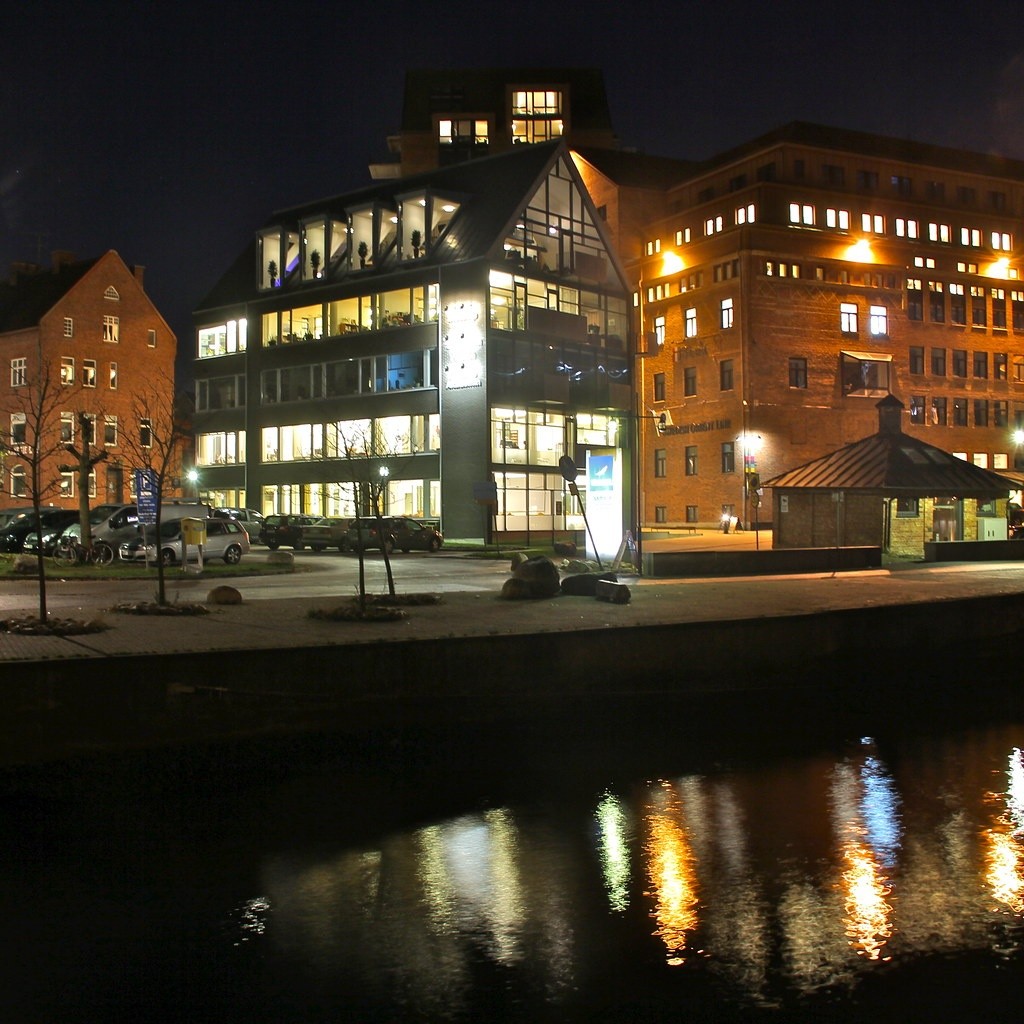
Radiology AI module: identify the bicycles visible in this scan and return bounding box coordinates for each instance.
[52,530,114,567]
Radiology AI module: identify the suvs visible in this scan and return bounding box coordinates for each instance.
[347,516,444,554]
[259,513,327,550]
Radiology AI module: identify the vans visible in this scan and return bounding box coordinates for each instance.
[211,507,268,545]
[118,516,251,566]
[1,506,59,533]
[60,502,210,562]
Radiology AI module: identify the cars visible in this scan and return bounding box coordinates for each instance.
[299,518,358,554]
[21,515,86,556]
[0,509,78,554]
